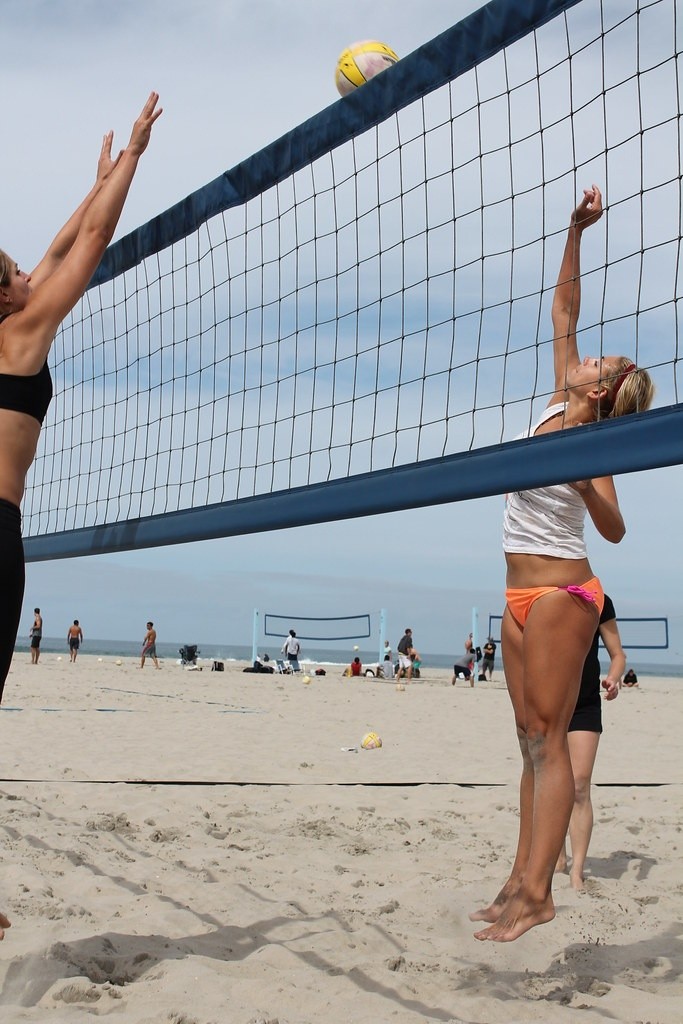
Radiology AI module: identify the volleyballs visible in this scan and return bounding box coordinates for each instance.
[333,38,401,98]
[56,656,63,662]
[360,731,383,751]
[115,659,122,666]
[301,675,311,685]
[353,645,360,651]
[97,657,103,662]
[395,684,405,692]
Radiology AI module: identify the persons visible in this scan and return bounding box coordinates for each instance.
[0,92,163,939]
[281,630,300,660]
[351,657,362,677]
[377,641,394,678]
[256,654,269,664]
[67,620,83,662]
[622,669,638,687]
[395,628,421,682]
[136,622,161,669]
[28,608,43,664]
[483,637,497,680]
[315,667,326,675]
[452,633,477,687]
[467,183,654,943]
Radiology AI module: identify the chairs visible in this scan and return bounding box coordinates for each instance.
[179,643,201,670]
[287,659,305,675]
[273,658,294,675]
